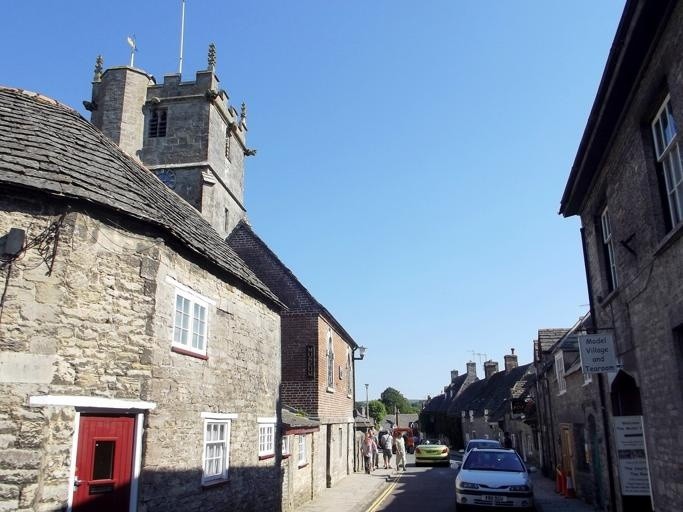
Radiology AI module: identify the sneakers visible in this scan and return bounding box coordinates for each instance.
[403,467,407,471]
[365,464,379,474]
[383,464,393,469]
[397,468,400,471]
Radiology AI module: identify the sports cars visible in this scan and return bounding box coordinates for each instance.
[414,439,450,467]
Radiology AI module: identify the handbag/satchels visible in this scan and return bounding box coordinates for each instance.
[381,444,384,449]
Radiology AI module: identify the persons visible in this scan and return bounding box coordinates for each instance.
[381,431,394,469]
[360,426,379,474]
[395,432,407,471]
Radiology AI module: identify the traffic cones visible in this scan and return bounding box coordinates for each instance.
[566,472,577,499]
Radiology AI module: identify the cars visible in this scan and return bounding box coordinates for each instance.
[449,446,537,512]
[459,439,502,463]
